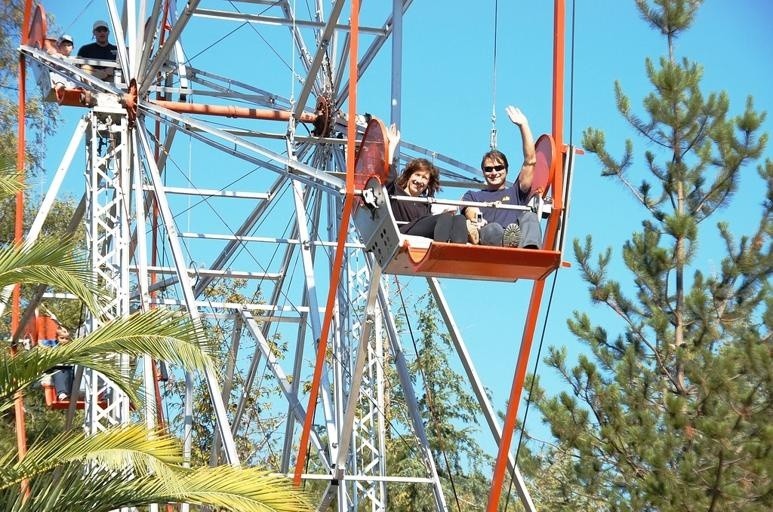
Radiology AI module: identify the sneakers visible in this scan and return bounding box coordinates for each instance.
[523,243,539,249]
[503,223,521,249]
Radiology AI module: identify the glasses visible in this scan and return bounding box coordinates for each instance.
[482,165,505,172]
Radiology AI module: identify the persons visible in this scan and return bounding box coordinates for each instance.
[77,19,129,90]
[44,34,92,105]
[460,105,541,250]
[383,123,467,243]
[41,324,74,401]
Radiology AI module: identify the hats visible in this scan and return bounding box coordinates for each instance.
[93,21,109,32]
[57,34,74,46]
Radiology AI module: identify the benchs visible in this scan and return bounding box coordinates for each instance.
[32,339,108,409]
[352,175,562,283]
[34,42,142,109]
